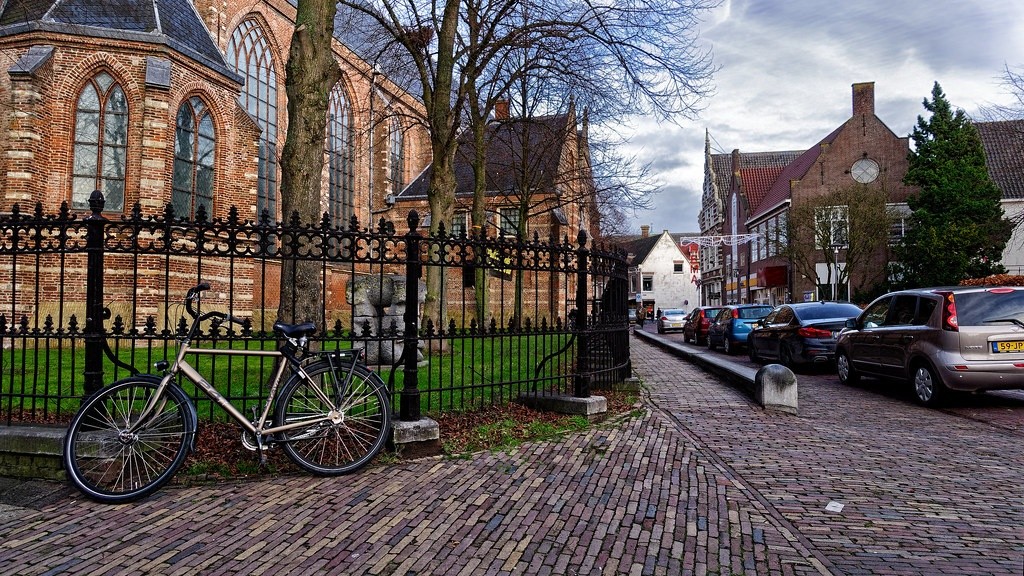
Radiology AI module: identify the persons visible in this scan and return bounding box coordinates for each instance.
[636,303,646,330]
[655,307,661,321]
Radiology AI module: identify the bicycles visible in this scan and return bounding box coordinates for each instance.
[63,282,392,505]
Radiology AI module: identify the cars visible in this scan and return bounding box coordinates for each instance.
[656,309,688,334]
[746,299,869,373]
[628,308,638,322]
[835,286,1024,410]
[683,306,729,347]
[707,303,777,357]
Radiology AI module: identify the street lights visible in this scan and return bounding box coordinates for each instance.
[830,241,845,302]
